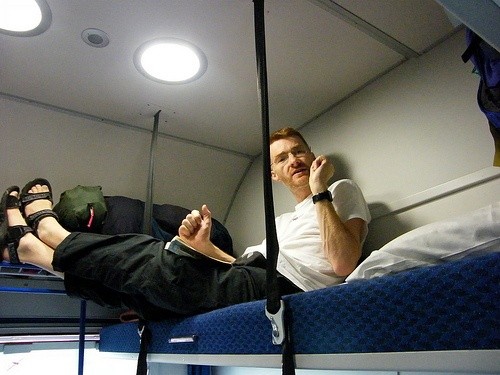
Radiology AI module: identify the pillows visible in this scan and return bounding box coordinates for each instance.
[345,201,500,282]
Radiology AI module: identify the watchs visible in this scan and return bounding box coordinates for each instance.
[312,190,333,204]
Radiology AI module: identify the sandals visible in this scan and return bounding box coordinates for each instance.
[0,185,34,266]
[19,178,60,231]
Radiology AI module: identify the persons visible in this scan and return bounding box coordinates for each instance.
[0,127,371,321]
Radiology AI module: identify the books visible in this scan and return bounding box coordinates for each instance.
[164,236,232,265]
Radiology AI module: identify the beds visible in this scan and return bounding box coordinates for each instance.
[99,251,500,371]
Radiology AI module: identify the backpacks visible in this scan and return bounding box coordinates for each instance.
[53,185,234,256]
